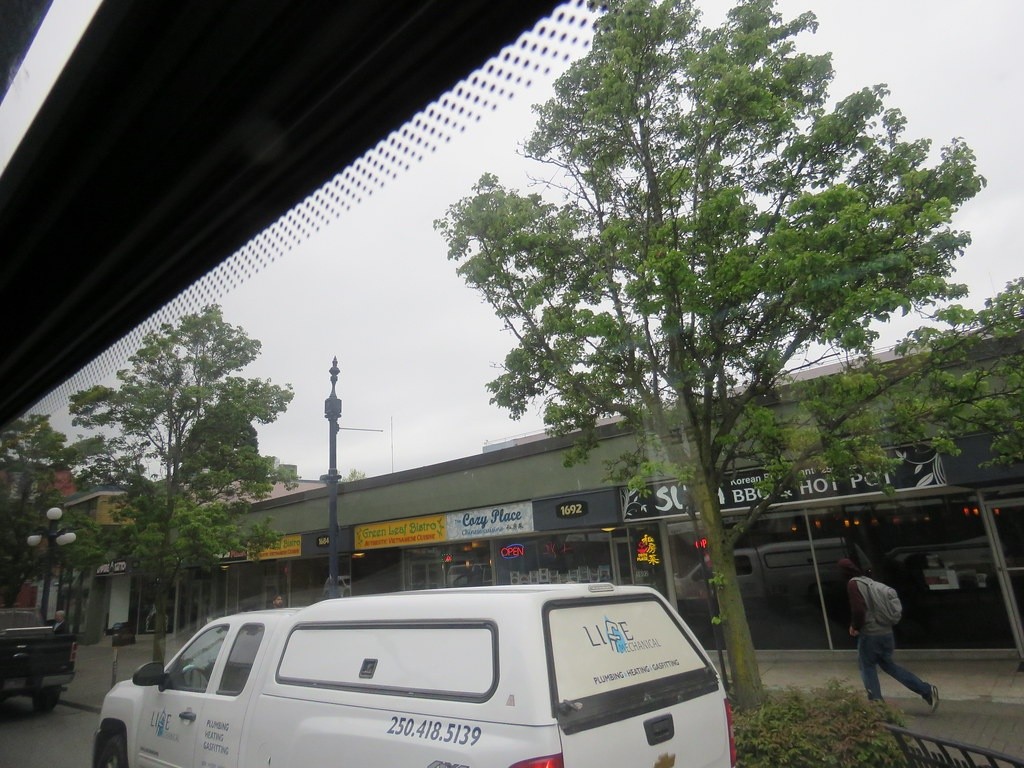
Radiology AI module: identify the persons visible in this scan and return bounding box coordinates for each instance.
[840,559,941,713]
[51,611,66,636]
[269,595,286,609]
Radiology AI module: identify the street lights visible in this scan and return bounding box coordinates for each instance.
[27,507,76,625]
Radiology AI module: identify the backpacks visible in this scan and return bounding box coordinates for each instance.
[849,576,902,626]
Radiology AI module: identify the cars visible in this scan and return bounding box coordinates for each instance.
[447,563,491,587]
[323,576,350,599]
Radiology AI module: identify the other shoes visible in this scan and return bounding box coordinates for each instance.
[62,687,67,691]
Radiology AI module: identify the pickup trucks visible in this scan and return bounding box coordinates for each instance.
[674,538,872,625]
[93,583,737,768]
[0,607,79,715]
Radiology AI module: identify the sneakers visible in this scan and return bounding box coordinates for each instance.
[929,685,940,713]
